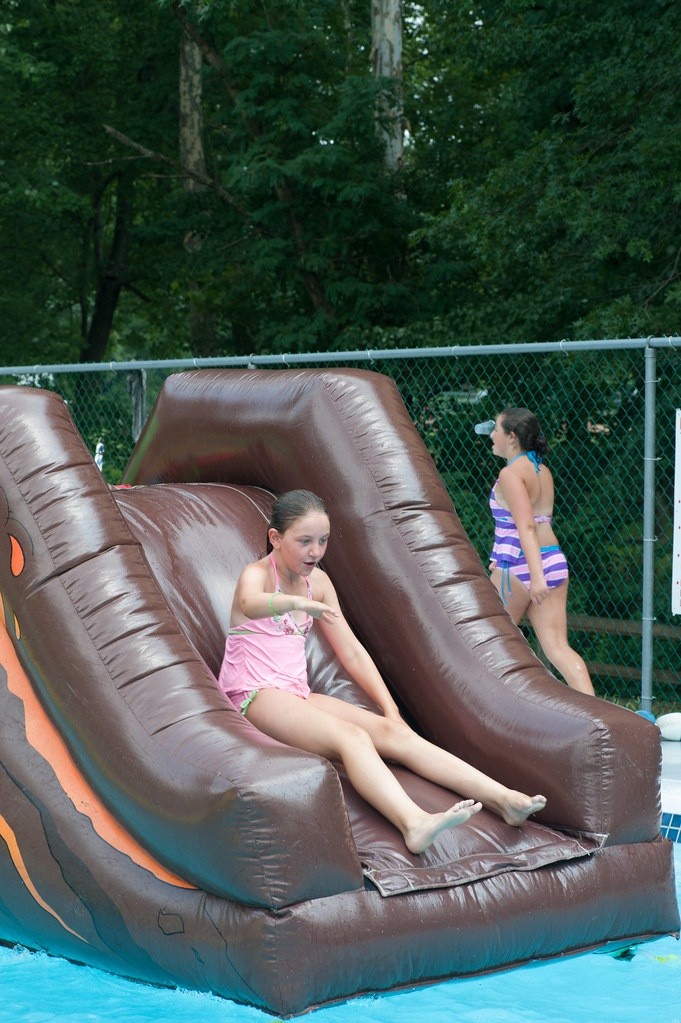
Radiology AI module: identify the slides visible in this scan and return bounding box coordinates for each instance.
[2,370,678,1014]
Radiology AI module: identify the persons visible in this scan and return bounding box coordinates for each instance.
[485,405,596,699]
[212,488,547,857]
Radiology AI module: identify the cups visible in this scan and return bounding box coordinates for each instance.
[474,419,494,435]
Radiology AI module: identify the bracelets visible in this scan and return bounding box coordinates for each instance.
[267,591,286,622]
